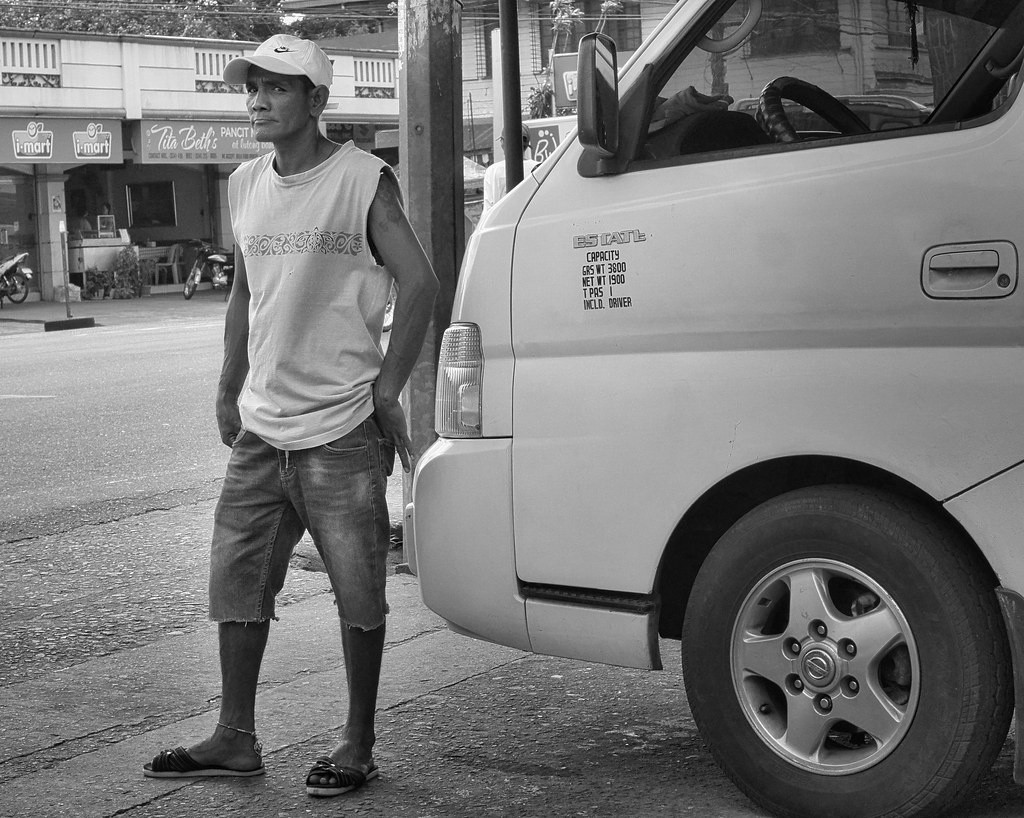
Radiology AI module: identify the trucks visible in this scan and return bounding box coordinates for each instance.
[404,0,1024,818]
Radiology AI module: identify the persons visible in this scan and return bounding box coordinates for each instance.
[484,123,541,211]
[142,33,439,795]
[71,202,111,231]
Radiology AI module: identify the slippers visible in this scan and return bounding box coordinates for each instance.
[142,746,265,777]
[306,755,379,795]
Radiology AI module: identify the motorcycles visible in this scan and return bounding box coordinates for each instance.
[183,236,232,300]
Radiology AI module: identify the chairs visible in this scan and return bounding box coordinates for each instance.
[156,243,183,284]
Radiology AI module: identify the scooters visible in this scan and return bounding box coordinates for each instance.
[0,250,34,305]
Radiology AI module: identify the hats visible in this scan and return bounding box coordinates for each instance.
[223,35,333,90]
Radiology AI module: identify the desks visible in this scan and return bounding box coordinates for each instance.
[139,246,184,284]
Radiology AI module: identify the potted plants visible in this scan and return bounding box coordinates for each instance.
[85,245,168,300]
